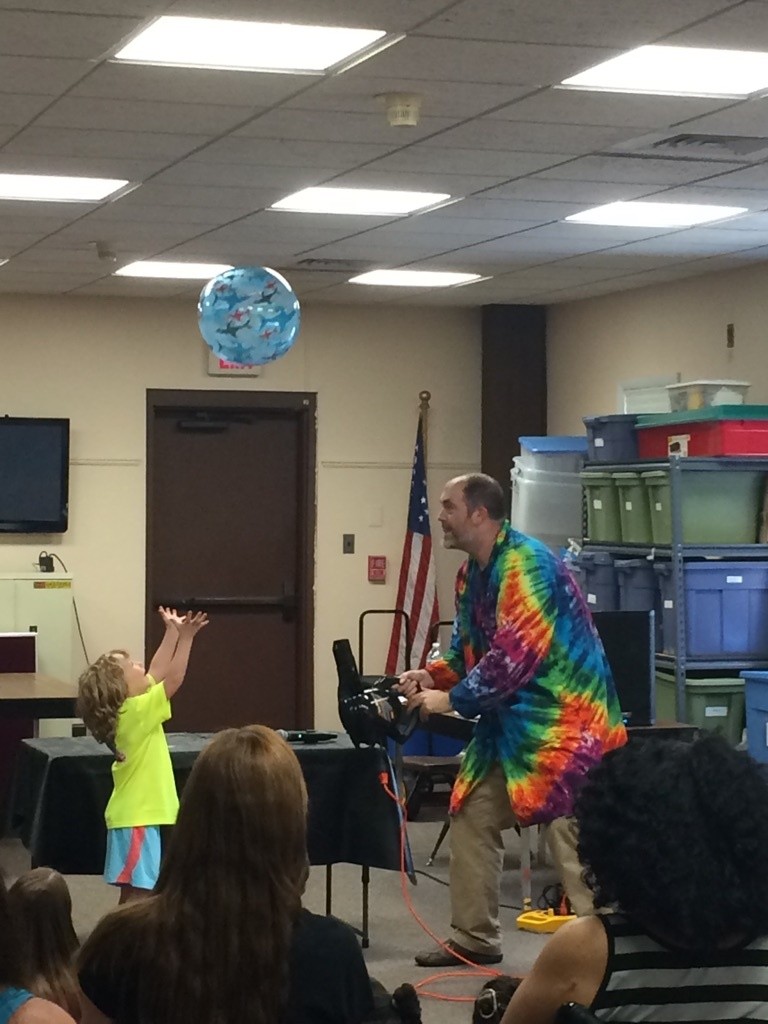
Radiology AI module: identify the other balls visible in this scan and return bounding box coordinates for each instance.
[195,266,307,367]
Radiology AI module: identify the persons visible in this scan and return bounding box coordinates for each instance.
[76,605,210,906]
[0,709,768,1024]
[391,474,627,969]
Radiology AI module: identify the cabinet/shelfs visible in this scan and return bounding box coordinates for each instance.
[579,450,768,723]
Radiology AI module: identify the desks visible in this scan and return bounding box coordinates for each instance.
[0,673,78,837]
[13,731,417,949]
[363,675,474,793]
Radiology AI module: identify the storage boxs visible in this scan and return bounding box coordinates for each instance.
[511,378,768,762]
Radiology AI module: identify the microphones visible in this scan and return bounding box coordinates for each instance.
[273,729,338,744]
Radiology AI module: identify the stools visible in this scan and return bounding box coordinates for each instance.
[400,754,463,865]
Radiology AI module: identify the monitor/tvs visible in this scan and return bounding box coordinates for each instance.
[0,417,69,531]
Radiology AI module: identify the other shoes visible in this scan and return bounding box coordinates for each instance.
[415,938,502,967]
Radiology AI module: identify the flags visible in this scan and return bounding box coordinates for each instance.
[382,413,443,680]
